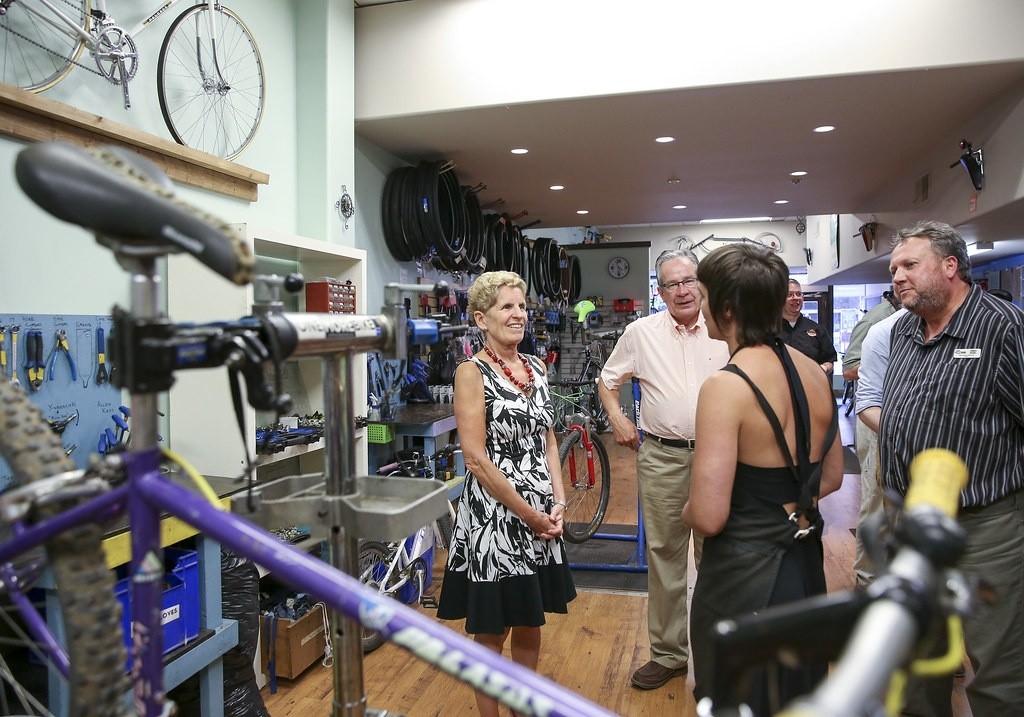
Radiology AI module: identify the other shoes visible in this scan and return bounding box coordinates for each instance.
[630,660,688,689]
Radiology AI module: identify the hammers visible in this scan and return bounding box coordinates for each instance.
[367,356,374,392]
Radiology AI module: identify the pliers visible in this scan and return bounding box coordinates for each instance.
[49,329,77,381]
[24,331,46,392]
[375,370,385,397]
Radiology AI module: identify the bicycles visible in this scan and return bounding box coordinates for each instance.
[838,351,859,418]
[326,443,462,653]
[0,0,267,166]
[0,135,975,716]
[547,378,611,544]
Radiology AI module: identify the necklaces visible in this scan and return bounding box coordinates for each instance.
[482,344,536,392]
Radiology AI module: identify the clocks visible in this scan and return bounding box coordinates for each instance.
[608,257,630,279]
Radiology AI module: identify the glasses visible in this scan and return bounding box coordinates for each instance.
[662,278,698,291]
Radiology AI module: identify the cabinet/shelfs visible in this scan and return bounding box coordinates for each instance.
[166,225,369,690]
[8,470,265,717]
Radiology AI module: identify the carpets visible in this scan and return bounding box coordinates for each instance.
[563,522,647,592]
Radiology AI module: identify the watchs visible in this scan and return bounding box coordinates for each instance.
[554,500,569,513]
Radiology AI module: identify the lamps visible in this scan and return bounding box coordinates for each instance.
[967,243,994,256]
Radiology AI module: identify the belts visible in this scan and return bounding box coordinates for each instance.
[648,434,694,450]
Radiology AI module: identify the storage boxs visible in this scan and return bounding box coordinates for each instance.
[114,545,198,676]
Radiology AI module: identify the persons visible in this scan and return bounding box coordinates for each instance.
[678,241,847,717]
[842,283,914,588]
[781,276,838,375]
[597,247,732,692]
[449,268,569,717]
[875,218,1024,717]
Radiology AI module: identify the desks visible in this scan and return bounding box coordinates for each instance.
[391,401,466,501]
